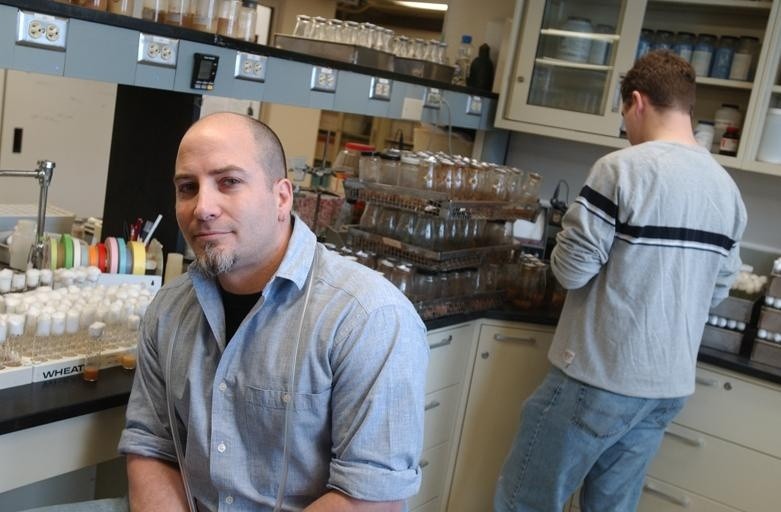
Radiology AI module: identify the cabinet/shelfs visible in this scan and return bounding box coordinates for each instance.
[569,363,781,512]
[446,323,558,511]
[326,178,542,324]
[412,321,478,511]
[493,0,781,177]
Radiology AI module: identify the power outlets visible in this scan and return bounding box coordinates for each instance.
[233,50,268,82]
[424,86,447,109]
[368,76,394,101]
[311,66,339,92]
[14,9,70,51]
[137,34,181,67]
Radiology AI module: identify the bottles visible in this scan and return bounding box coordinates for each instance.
[292,14,447,66]
[451,35,493,95]
[323,241,547,302]
[694,103,744,157]
[359,204,517,252]
[555,15,761,82]
[52,0,257,45]
[0,265,157,382]
[358,148,543,204]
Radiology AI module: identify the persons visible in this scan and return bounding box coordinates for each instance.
[117,112,430,512]
[492,49,748,512]
